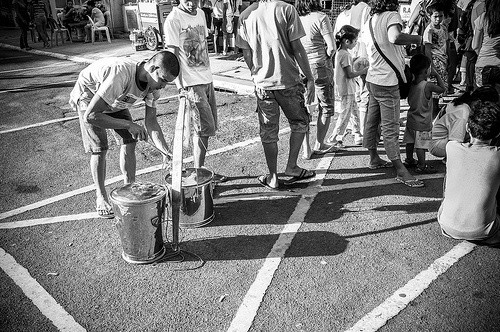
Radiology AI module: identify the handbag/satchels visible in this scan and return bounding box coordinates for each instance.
[396,64,413,99]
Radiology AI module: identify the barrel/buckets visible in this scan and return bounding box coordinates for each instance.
[165,168,215,229]
[110,183,166,263]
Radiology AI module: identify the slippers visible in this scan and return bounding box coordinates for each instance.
[283,168,316,185]
[395,173,425,188]
[95,203,115,219]
[314,144,340,154]
[367,160,394,170]
[257,175,279,190]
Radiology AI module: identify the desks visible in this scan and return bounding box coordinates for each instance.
[68,22,88,42]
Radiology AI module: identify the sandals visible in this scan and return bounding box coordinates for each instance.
[403,158,418,168]
[413,164,439,175]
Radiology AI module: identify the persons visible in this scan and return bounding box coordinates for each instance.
[164,1,229,184]
[437,100,500,240]
[68,52,180,220]
[236,0,316,189]
[13,0,500,188]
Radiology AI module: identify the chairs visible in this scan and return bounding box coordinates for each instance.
[47,17,73,47]
[87,15,111,46]
